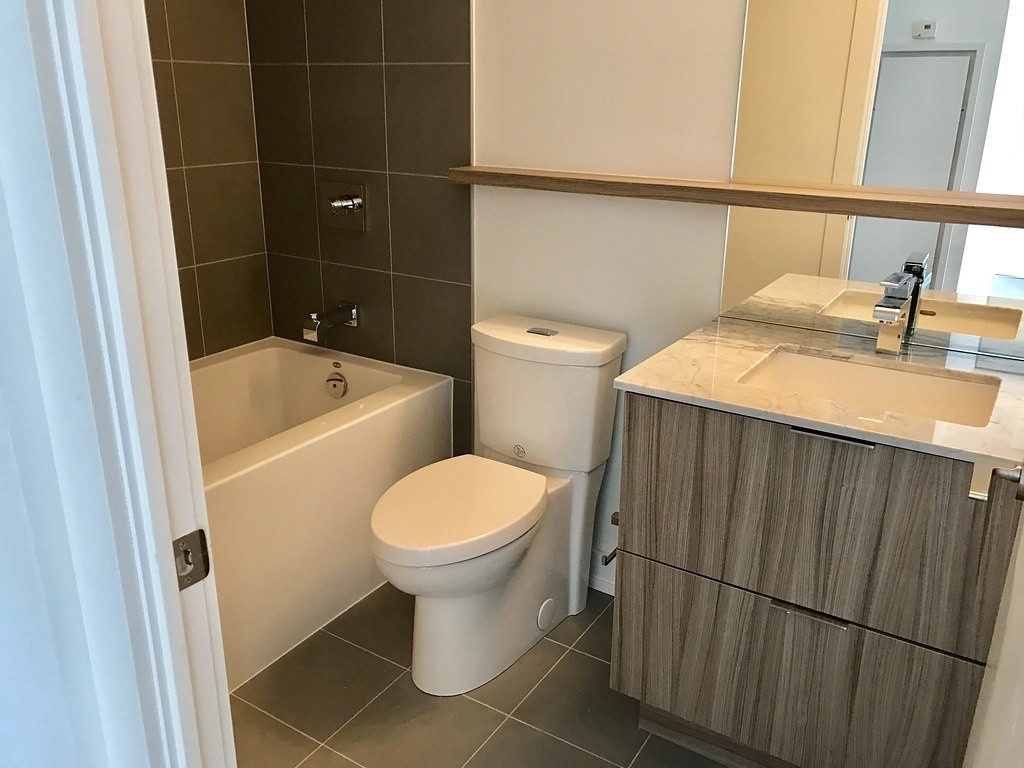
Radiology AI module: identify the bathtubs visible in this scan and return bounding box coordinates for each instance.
[188,335,453,696]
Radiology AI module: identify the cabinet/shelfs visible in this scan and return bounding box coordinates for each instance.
[610,389,1024,768]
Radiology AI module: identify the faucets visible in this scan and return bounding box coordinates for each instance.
[900,252,933,336]
[302,300,359,343]
[872,271,914,356]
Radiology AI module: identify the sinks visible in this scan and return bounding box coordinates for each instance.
[734,343,1002,428]
[816,288,1022,341]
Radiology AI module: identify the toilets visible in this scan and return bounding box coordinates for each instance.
[369,312,627,697]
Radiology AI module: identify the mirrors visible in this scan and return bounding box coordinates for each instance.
[718,1,1023,362]
